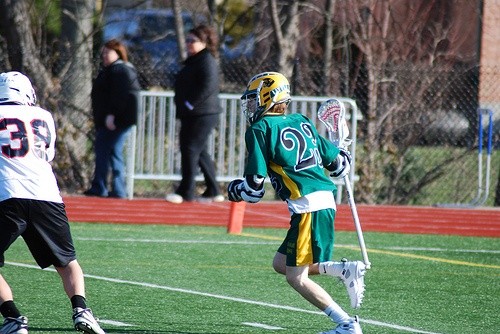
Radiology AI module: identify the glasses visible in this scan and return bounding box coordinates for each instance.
[186,39,202,43]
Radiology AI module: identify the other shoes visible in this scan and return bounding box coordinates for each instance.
[166,193,184,204]
[108,191,118,197]
[83,188,98,196]
[196,194,224,203]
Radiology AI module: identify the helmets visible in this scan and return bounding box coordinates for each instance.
[241,72,291,125]
[0,72,37,106]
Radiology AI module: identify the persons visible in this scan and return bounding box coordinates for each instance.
[84,38,139,201]
[0,72,105,334]
[165,24,225,204]
[228,71,366,334]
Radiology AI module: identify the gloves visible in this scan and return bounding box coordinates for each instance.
[227,180,243,202]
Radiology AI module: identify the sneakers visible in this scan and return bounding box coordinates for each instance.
[318,315,362,334]
[72,307,105,334]
[341,258,365,310]
[0,316,29,334]
